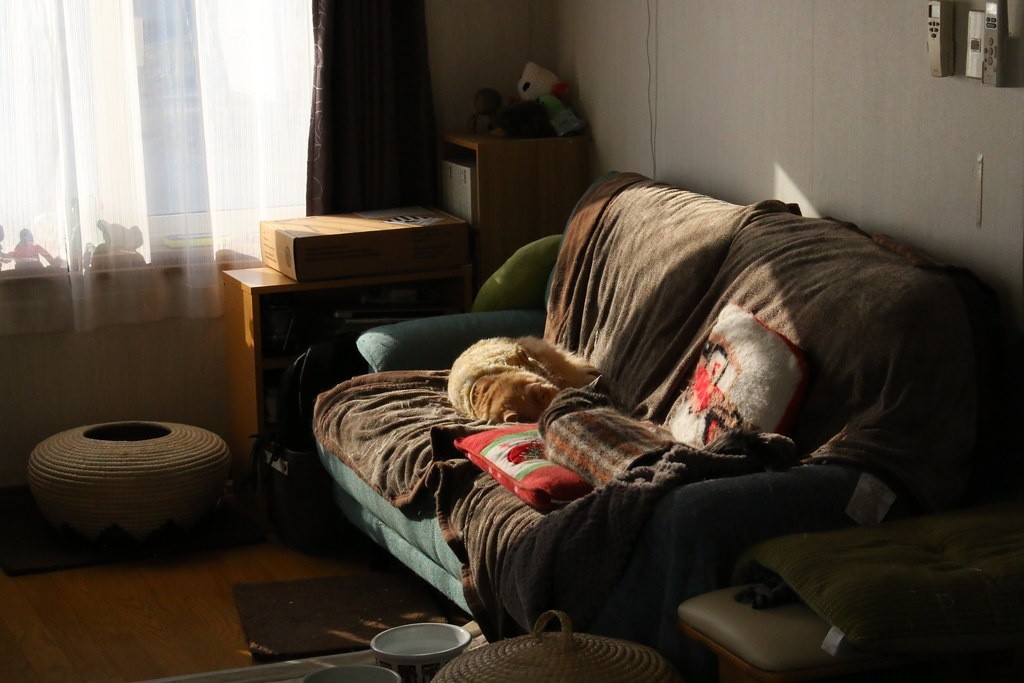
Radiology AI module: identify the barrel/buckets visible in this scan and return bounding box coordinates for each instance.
[264,433,340,556]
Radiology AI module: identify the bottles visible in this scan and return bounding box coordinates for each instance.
[261,302,300,357]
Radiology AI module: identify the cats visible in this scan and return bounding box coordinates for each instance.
[538,374,768,489]
[447,334,605,426]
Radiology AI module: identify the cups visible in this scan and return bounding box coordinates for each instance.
[370,622,472,683]
[302,664,402,683]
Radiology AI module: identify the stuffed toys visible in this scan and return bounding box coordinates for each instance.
[516,58,570,103]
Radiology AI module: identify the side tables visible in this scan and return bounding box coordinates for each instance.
[221,127,587,487]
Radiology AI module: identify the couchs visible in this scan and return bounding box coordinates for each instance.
[297,171,1011,629]
